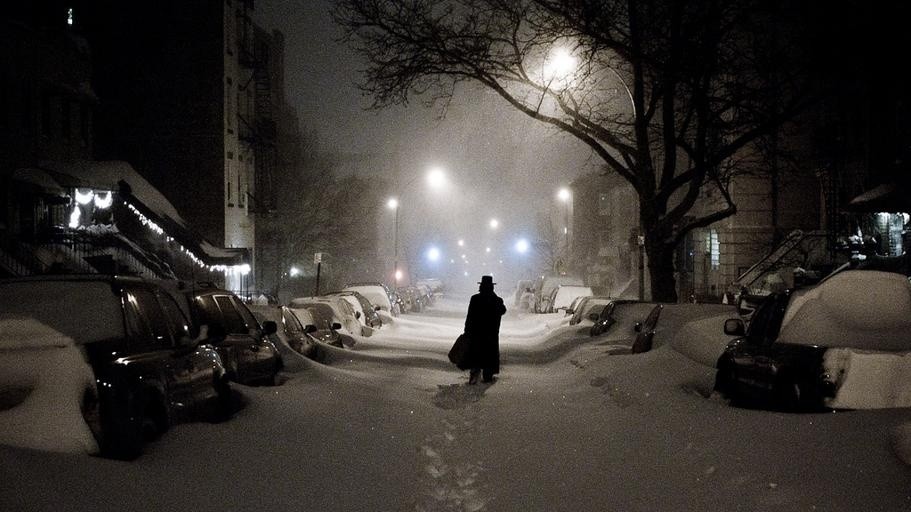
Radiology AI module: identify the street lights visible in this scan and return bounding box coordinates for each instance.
[385,166,446,292]
[557,188,573,267]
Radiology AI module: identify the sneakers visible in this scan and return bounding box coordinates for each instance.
[468,369,498,383]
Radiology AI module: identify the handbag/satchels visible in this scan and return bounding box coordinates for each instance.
[449,332,473,371]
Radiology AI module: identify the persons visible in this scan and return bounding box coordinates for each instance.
[462,275,508,385]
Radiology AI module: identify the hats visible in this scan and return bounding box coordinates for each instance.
[477,275,498,286]
